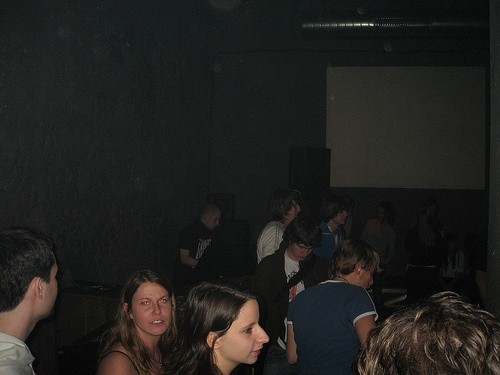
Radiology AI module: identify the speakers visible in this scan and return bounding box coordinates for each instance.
[288,146,331,193]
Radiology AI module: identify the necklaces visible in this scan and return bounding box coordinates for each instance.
[151,356,164,366]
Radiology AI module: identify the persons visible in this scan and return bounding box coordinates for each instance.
[164,282,269,375]
[96,265,177,375]
[250,216,333,375]
[0,226,59,375]
[175,186,500,311]
[286,237,380,375]
